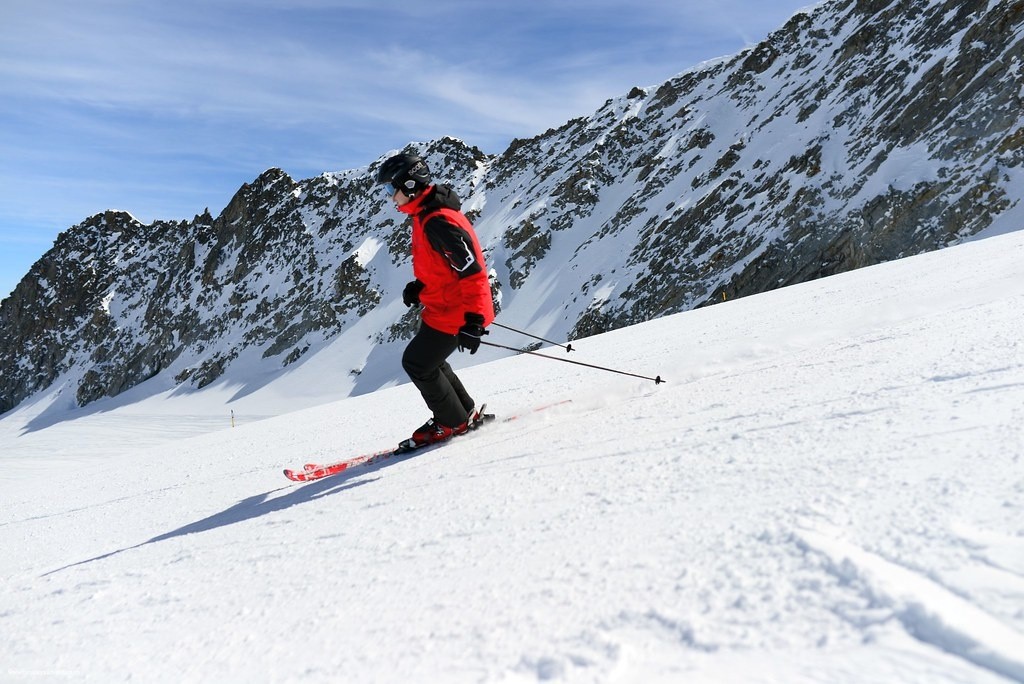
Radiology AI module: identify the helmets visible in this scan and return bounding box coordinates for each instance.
[376,152,432,185]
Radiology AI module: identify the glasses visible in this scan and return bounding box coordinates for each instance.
[385,179,397,197]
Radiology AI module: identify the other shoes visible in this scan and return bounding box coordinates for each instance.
[411,410,479,443]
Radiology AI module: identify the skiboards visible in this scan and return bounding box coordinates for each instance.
[281,398,572,480]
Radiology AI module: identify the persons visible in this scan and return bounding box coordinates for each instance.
[373,153,499,451]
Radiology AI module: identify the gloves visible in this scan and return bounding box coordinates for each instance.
[457,313,487,353]
[402,280,424,306]
